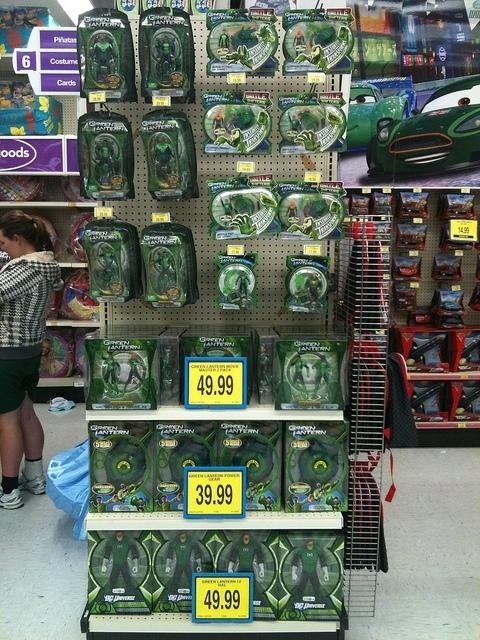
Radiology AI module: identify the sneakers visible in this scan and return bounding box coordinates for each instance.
[18,468,48,495]
[0,481,26,510]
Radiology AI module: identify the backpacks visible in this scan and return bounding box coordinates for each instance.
[352,345,420,453]
[347,217,393,332]
[342,458,391,575]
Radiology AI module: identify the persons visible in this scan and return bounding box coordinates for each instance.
[90,31,177,85]
[101,532,329,606]
[92,242,176,296]
[92,134,176,188]
[74,4,353,622]
[101,344,331,396]
[0,209,66,511]
[212,26,321,303]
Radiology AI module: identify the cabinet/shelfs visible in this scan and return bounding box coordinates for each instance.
[339,190,479,452]
[0,0,93,396]
[77,4,350,640]
